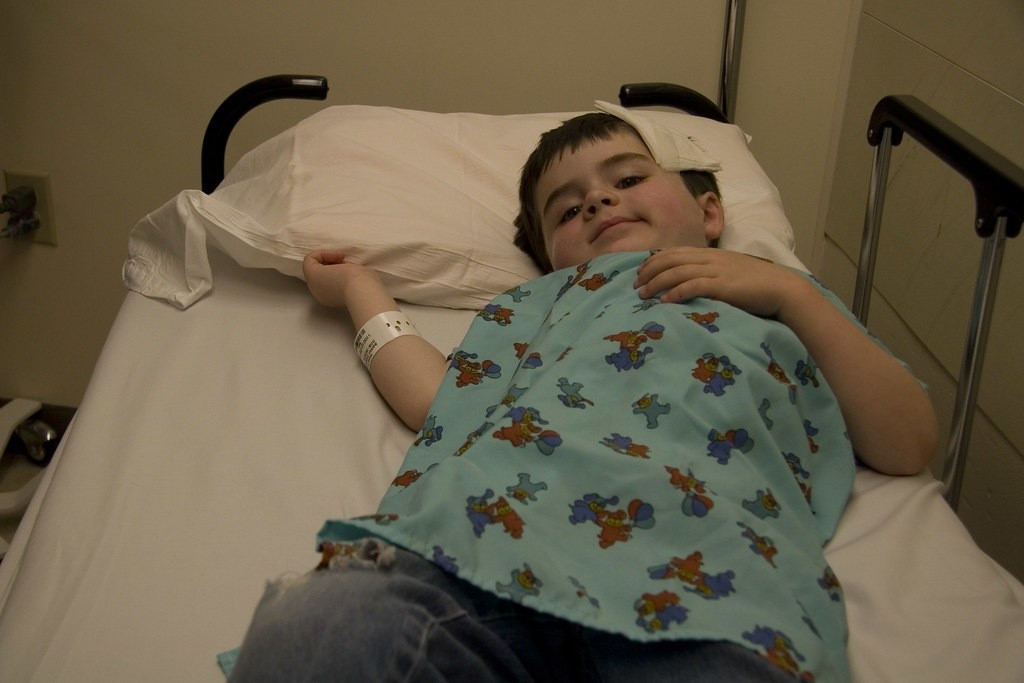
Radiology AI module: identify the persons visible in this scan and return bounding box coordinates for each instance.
[228,113,937,683]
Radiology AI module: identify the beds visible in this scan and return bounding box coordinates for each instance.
[0,77,1024,683]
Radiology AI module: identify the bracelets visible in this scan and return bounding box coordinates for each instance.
[354,311,421,372]
